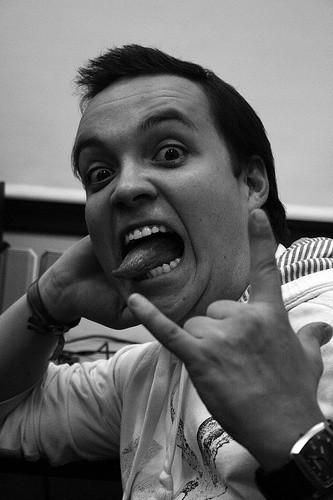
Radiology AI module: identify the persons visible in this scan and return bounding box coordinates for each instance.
[0,41,333,500]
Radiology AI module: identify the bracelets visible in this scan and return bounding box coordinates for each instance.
[22,280,81,339]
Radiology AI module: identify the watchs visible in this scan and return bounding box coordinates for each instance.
[252,415,333,500]
